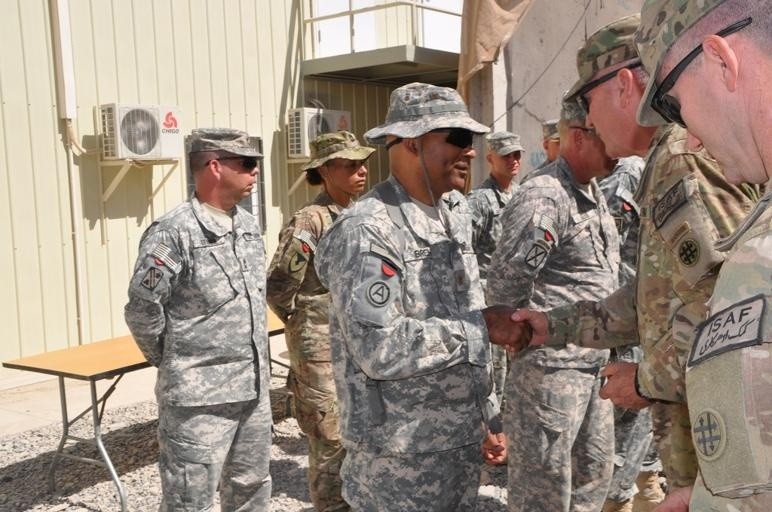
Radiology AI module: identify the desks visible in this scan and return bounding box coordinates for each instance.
[1,304,293,512]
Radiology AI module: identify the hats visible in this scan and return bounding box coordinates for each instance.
[634,0,729,128]
[301,131,376,170]
[561,14,643,103]
[486,132,526,156]
[364,83,491,144]
[542,121,559,139]
[190,128,264,158]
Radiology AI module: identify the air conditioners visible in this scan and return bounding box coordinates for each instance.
[100,100,186,161]
[287,106,352,159]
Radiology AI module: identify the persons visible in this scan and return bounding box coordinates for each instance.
[520,120,561,184]
[597,154,664,512]
[633,0,772,512]
[504,12,762,494]
[313,81,534,512]
[124,128,272,512]
[480,90,622,511]
[466,129,527,409]
[266,130,376,512]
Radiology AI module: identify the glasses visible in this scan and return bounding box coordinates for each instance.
[205,156,257,168]
[649,17,755,128]
[576,62,642,116]
[445,129,473,148]
